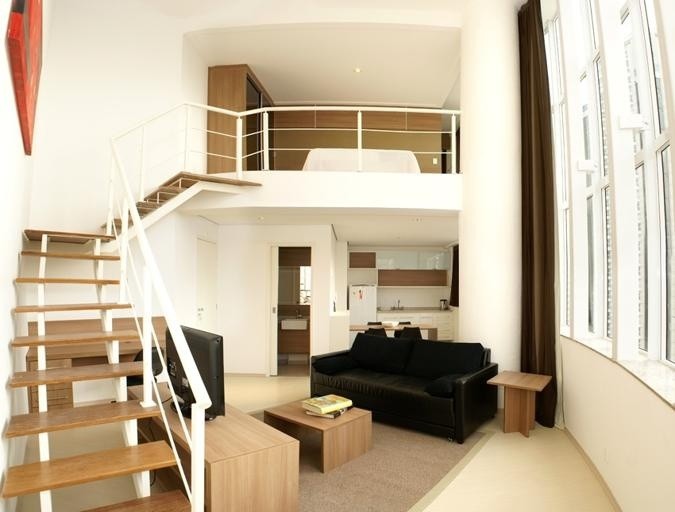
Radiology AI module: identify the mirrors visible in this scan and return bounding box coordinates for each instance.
[279,265,312,306]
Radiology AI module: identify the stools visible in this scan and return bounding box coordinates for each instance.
[487,370,553,440]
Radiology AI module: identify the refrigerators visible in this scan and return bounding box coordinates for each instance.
[349,283,377,348]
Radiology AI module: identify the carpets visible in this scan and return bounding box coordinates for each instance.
[245,396,496,511]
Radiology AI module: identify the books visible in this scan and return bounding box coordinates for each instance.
[301,393,354,414]
[305,405,353,420]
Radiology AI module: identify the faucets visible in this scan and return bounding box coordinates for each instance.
[398,299,400,308]
[296,309,302,319]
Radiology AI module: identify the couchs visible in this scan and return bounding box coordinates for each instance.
[310,332,498,444]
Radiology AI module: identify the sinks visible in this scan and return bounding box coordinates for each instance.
[281,319,308,331]
[377,307,403,311]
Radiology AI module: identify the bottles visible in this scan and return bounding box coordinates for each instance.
[439,298,448,310]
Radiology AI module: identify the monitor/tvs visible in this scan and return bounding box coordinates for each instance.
[165,325,225,422]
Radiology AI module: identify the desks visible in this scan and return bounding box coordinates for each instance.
[117,380,301,512]
